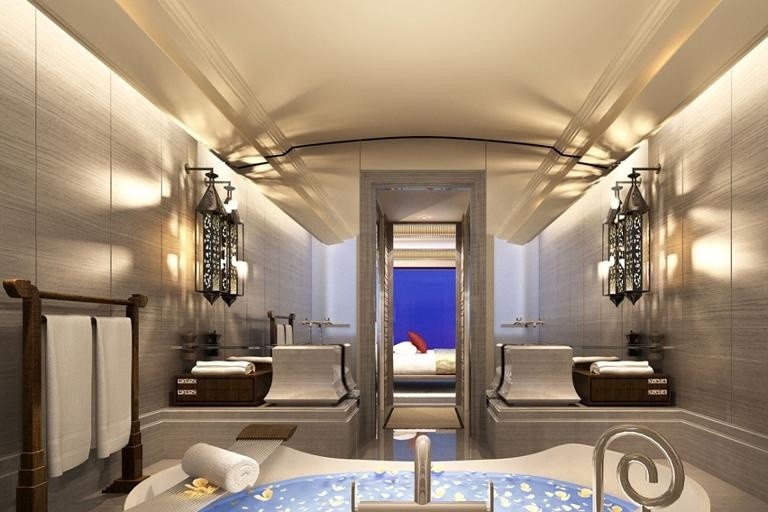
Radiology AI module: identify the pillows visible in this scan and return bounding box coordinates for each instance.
[407,331,428,353]
[392,341,417,353]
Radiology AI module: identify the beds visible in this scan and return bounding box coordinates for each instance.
[392,348,457,383]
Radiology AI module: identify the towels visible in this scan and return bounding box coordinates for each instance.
[276,324,285,347]
[181,441,260,494]
[44,315,92,478]
[572,356,622,368]
[226,356,273,363]
[284,324,294,346]
[589,360,654,374]
[93,316,133,459]
[190,361,255,375]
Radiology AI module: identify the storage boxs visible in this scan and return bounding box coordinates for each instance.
[173,369,273,406]
[572,369,672,407]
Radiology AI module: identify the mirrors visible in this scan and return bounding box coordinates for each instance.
[485,139,642,398]
[361,138,485,172]
[205,136,361,398]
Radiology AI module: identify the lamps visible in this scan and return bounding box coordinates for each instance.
[617,162,661,305]
[204,177,245,308]
[183,163,229,307]
[601,178,644,308]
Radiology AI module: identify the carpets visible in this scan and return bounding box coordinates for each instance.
[381,406,464,429]
